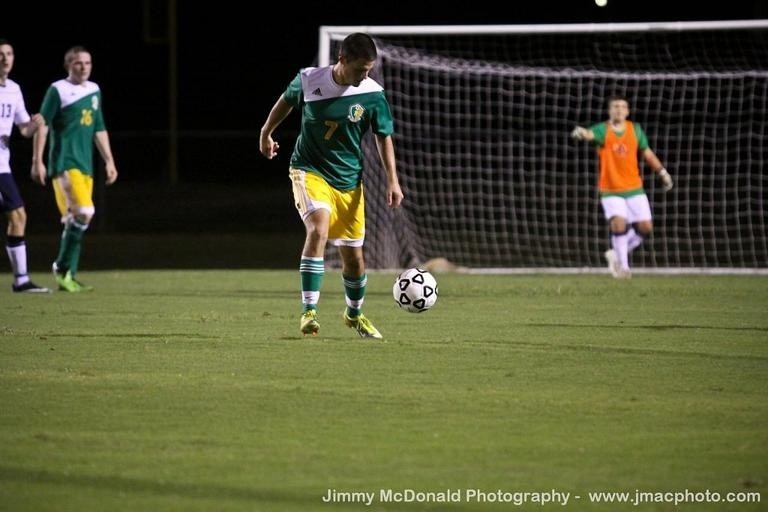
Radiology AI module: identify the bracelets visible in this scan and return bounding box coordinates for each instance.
[657,166,666,179]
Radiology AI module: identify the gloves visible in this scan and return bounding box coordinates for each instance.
[571,126,587,140]
[659,168,673,193]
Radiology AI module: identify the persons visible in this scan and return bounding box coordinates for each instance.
[259,32,404,342]
[28,43,121,296]
[0,40,58,295]
[568,94,674,280]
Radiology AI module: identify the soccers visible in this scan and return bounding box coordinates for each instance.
[392,266,438,312]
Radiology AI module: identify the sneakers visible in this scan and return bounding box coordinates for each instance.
[603,249,632,281]
[299,309,320,336]
[342,306,383,339]
[12,281,53,293]
[51,261,94,293]
